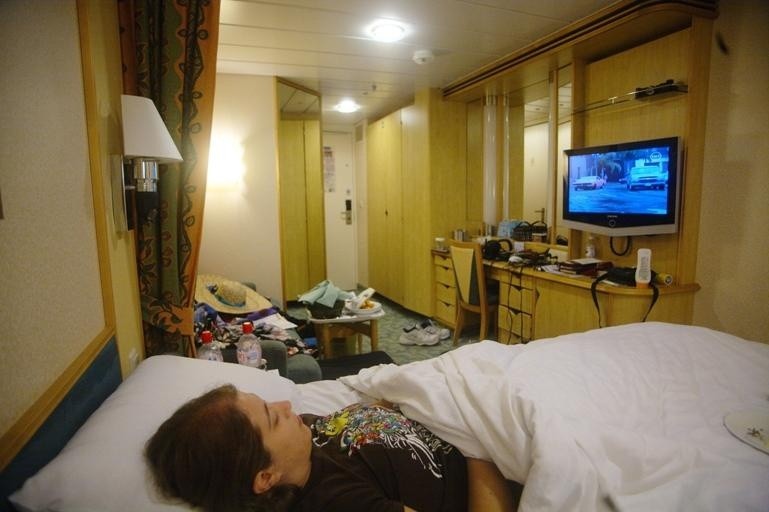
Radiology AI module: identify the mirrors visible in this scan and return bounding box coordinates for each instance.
[506,63,572,237]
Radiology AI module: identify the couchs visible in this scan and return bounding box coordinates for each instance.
[202,280,324,384]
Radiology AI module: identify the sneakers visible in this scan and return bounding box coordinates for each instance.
[398,326,440,346]
[416,318,451,340]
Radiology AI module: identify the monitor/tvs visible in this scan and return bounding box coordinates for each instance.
[557,136,680,237]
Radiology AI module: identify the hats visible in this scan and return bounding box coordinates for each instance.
[196,274,273,316]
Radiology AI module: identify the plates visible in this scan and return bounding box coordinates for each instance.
[346,303,382,314]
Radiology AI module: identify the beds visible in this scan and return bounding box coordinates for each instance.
[0,321,769,512]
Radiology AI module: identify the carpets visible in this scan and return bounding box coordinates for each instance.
[317,350,396,380]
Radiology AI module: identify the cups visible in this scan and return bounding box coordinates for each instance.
[434,237,445,251]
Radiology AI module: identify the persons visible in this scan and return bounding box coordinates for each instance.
[141,381,525,512]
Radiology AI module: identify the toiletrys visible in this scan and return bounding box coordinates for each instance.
[634,249,651,289]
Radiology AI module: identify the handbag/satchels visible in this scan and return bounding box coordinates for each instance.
[605,266,658,287]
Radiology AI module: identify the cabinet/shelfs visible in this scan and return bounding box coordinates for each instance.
[366,87,467,318]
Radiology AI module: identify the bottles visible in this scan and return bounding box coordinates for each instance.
[235,321,262,369]
[584,236,595,262]
[198,329,221,367]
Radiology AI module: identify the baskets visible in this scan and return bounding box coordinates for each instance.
[512,220,547,242]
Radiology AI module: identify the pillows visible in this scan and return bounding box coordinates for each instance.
[8,354,296,512]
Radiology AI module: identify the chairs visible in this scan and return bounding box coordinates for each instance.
[447,239,498,347]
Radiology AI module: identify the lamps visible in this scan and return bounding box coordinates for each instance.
[120,94,184,193]
[361,14,414,47]
[333,99,361,113]
[412,49,435,64]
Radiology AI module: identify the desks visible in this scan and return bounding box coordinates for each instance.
[431,248,701,346]
